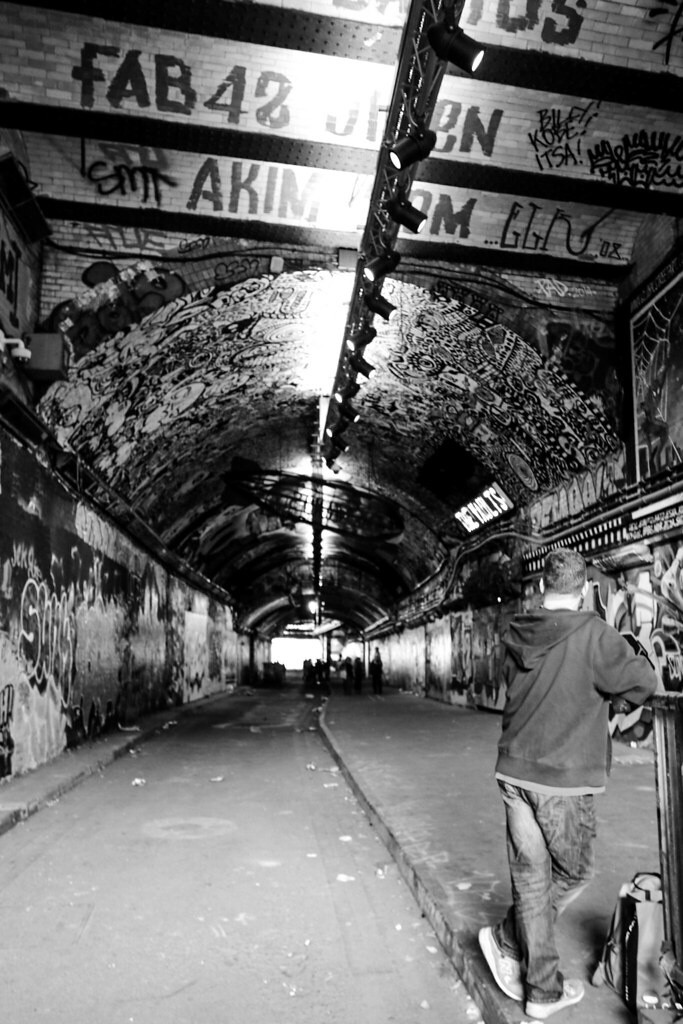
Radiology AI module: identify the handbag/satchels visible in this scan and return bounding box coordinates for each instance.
[593,873,669,1010]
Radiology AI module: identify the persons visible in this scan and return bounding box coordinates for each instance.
[478,549,656,1019]
[304,654,382,694]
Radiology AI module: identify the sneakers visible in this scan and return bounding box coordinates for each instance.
[479,927,525,1001]
[526,979,585,1020]
